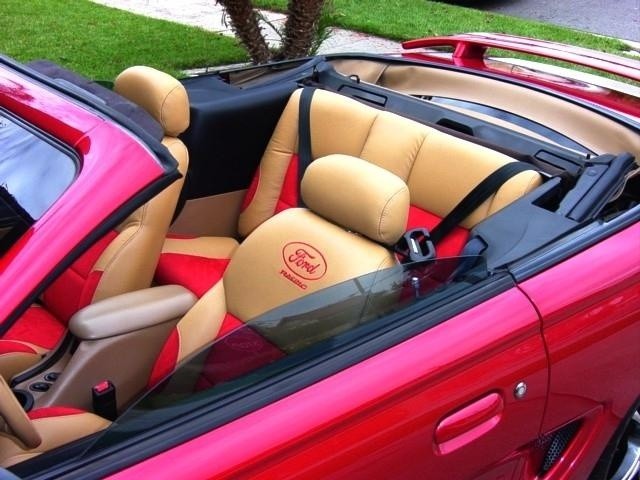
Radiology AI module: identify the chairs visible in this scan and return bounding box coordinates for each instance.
[0,155,411,467]
[0,64,191,373]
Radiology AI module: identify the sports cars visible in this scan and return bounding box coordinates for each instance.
[0,31,640,480]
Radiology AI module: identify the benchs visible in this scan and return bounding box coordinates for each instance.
[158,86,543,325]
